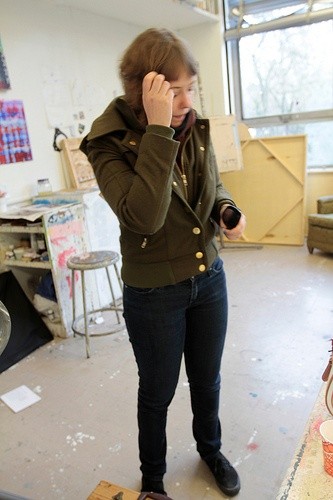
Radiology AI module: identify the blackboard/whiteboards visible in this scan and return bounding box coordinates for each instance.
[205,114,245,174]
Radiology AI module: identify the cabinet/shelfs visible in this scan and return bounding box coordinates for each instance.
[0,191,122,340]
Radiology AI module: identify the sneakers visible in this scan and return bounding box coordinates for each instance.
[198,451,240,496]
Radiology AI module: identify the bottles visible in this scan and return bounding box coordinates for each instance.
[36,179,53,196]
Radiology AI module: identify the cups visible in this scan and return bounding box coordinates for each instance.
[319,419,333,475]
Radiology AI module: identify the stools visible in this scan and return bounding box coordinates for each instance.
[66,251,127,359]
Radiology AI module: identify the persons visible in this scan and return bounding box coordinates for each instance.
[78,28,246,500]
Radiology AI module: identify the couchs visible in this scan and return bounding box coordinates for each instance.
[307,196,333,255]
